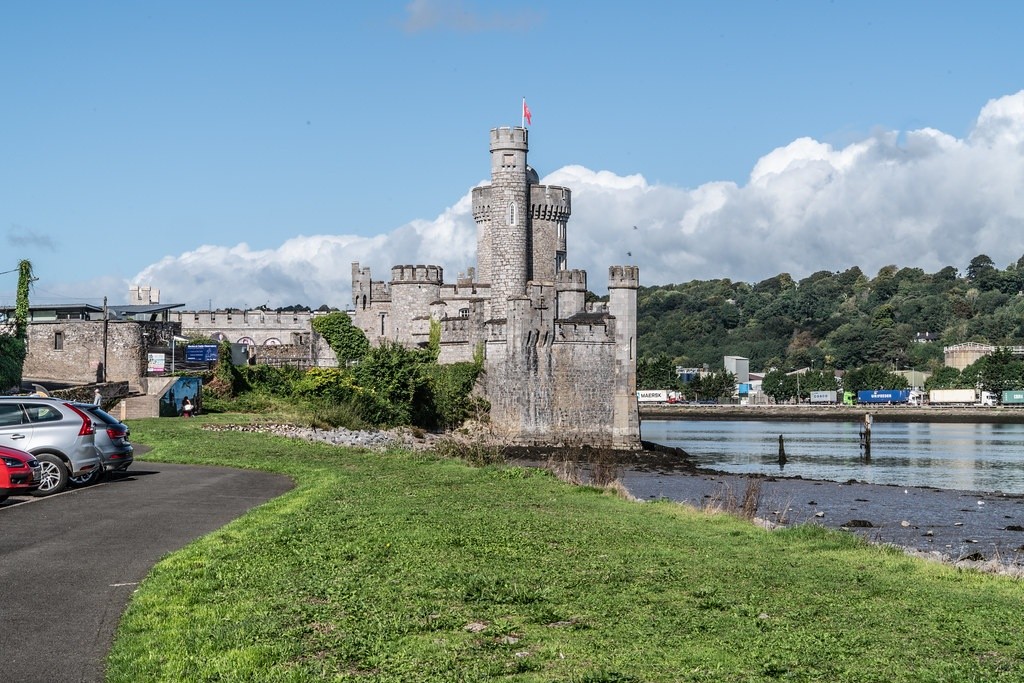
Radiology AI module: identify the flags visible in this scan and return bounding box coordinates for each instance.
[523,102,532,125]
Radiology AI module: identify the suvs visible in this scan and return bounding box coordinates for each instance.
[0,392,101,497]
[72,401,134,473]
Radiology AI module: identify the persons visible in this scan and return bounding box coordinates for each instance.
[169,389,200,418]
[93,389,102,409]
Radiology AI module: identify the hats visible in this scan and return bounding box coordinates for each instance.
[94,389,101,393]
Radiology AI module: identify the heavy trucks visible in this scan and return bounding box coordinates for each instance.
[636,389,682,404]
[1001,391,1024,409]
[857,390,919,408]
[928,388,996,407]
[808,389,857,405]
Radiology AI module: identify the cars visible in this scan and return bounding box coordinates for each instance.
[0,444,43,505]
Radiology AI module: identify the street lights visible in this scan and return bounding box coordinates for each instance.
[903,365,915,391]
[790,366,800,404]
